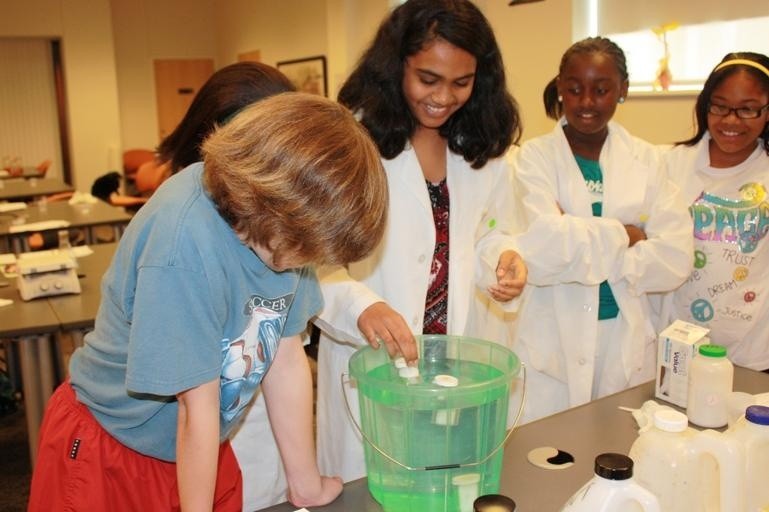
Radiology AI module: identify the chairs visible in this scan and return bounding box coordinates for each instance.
[122,150,174,195]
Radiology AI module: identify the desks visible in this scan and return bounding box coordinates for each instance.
[255,365,769,512]
[0,242,132,475]
[1,167,41,180]
[1,178,75,198]
[1,191,133,253]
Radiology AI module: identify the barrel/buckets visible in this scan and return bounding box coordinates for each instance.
[341,333,528,512]
[630,407,736,511]
[736,406,769,511]
[559,453,656,511]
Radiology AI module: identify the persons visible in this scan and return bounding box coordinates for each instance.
[505,37,699,427]
[646,49,769,375]
[302,1,528,482]
[160,59,312,511]
[25,89,390,512]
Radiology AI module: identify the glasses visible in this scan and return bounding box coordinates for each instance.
[708,100,769,119]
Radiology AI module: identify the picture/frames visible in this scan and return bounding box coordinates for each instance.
[278,55,328,96]
